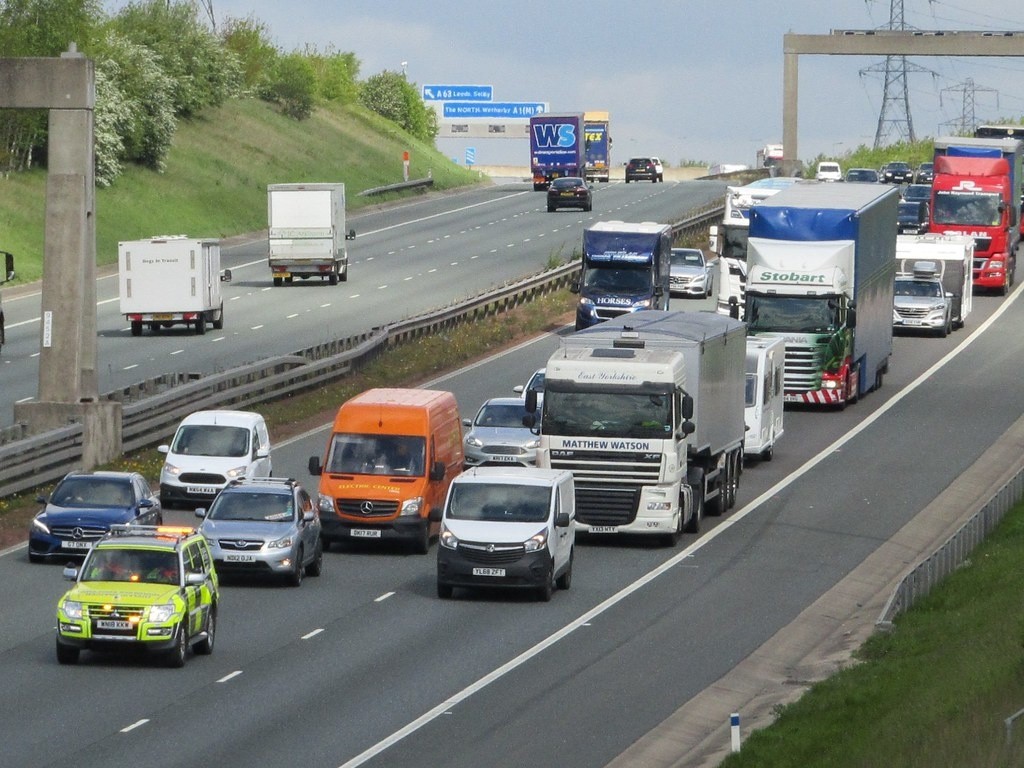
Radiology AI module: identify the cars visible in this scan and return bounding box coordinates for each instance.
[460,369,546,468]
[846,162,933,235]
[546,179,593,213]
[895,274,954,337]
[669,247,714,298]
[27,470,163,561]
[623,157,663,183]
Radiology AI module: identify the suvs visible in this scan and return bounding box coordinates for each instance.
[54,525,220,665]
[194,476,323,587]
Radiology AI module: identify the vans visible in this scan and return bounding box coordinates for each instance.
[308,386,462,555]
[157,410,271,507]
[815,162,842,181]
[430,468,576,600]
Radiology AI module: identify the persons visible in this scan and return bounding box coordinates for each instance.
[387,440,418,472]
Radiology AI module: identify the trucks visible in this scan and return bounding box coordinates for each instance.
[267,183,357,284]
[530,111,612,190]
[757,143,783,168]
[918,126,1024,295]
[118,234,232,333]
[572,219,671,332]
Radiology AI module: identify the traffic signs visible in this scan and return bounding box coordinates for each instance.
[422,85,546,118]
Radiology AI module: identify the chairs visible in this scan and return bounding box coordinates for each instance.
[102,553,140,581]
[492,406,509,424]
[645,160,650,164]
[142,552,180,586]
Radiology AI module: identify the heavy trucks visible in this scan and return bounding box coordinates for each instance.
[521,308,750,545]
[709,177,897,409]
